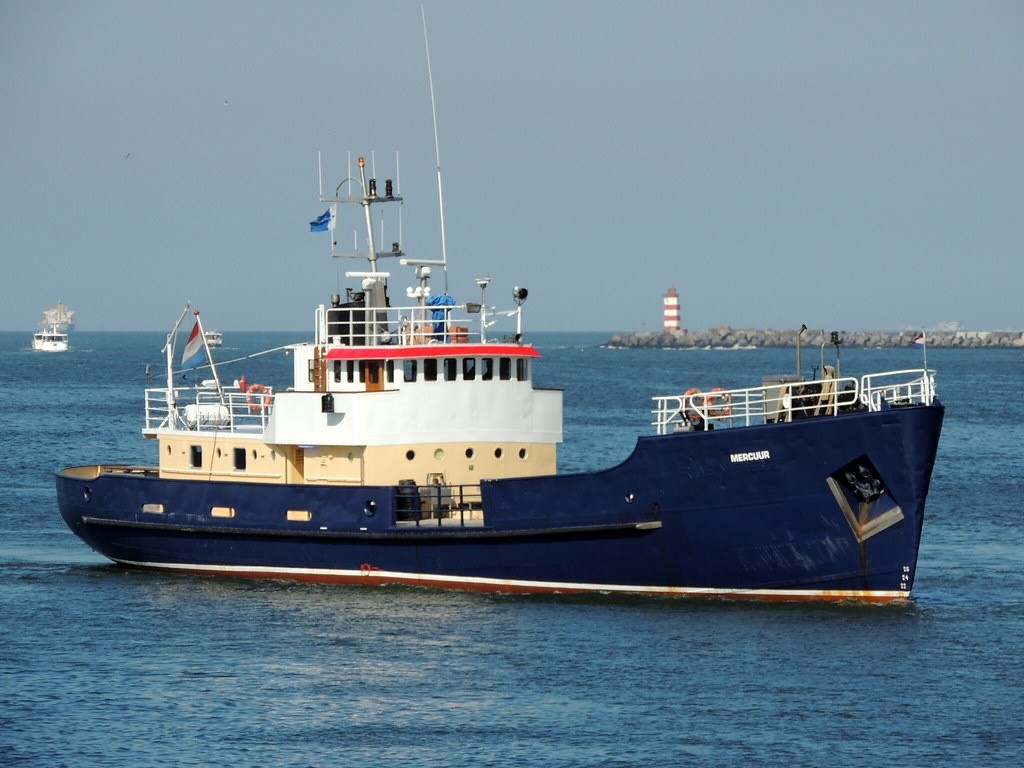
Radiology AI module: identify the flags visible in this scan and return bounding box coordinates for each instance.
[310,203,337,233]
[180,321,209,380]
[911,331,924,351]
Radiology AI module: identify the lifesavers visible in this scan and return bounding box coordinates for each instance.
[245,384,271,411]
[682,388,705,419]
[707,388,731,417]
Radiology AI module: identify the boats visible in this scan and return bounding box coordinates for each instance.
[44,304,75,335]
[34,327,67,353]
[204,330,223,347]
[55,145,944,609]
